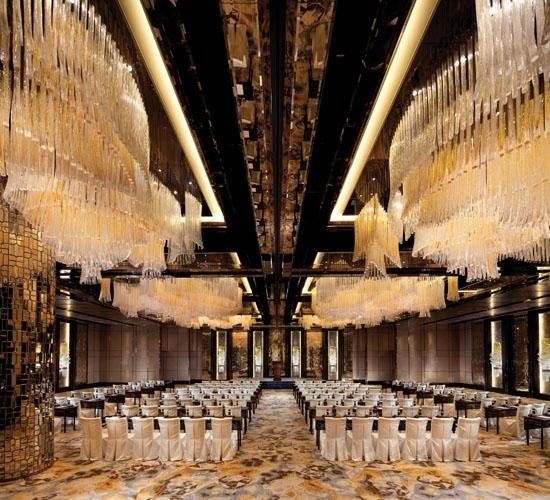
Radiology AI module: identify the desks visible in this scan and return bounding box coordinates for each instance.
[522,415,550,449]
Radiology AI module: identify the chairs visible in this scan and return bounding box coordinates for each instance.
[294,380,546,463]
[54,379,261,463]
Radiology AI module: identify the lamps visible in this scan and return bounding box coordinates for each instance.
[298,1,548,331]
[0,1,251,332]
